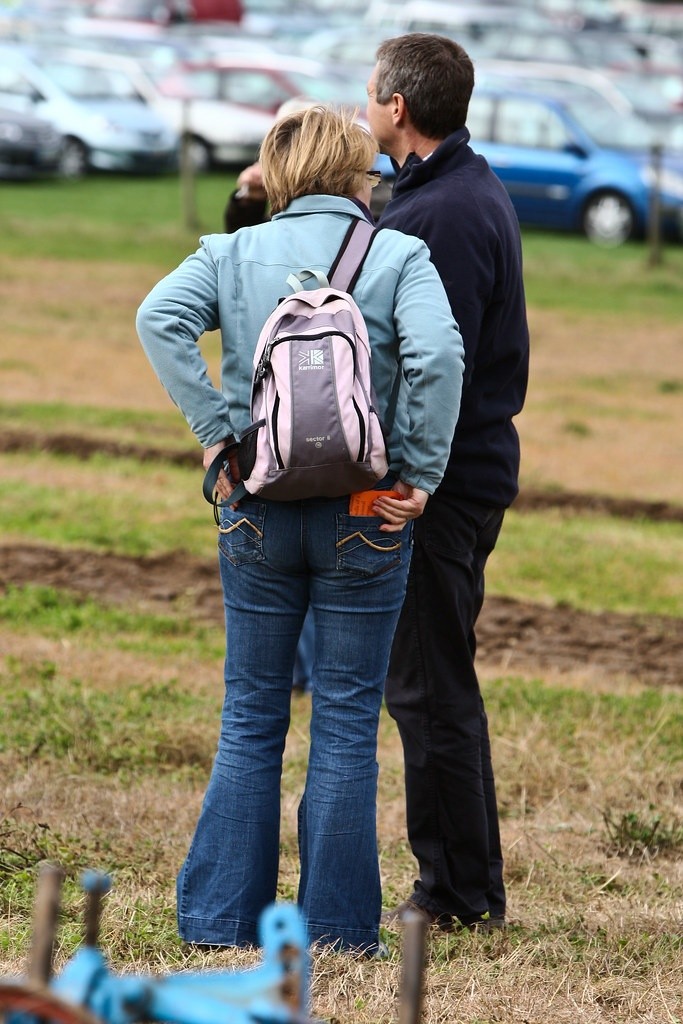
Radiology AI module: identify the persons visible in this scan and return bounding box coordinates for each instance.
[225,32,528,929]
[133,99,467,953]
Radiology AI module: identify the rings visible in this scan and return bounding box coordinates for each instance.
[406,516,408,522]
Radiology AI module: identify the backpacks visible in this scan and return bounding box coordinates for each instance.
[203,217,402,508]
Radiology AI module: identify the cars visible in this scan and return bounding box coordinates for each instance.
[0,0,683,254]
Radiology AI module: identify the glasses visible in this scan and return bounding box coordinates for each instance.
[357,169,381,188]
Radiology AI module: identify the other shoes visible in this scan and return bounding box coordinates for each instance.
[380,899,504,932]
